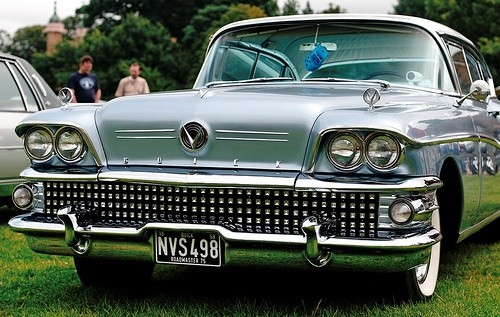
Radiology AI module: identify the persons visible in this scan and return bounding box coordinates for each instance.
[66,54,101,103]
[115,63,150,99]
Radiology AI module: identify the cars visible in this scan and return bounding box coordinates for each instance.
[8,14,500,301]
[0,54,62,199]
